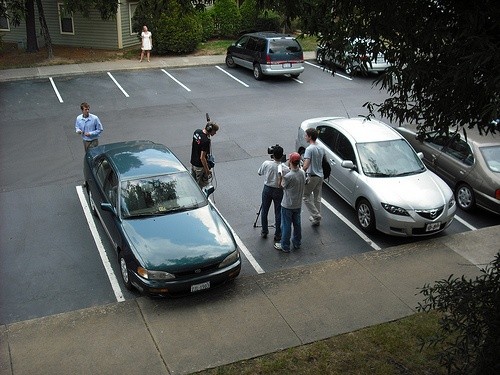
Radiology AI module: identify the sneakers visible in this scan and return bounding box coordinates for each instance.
[273,242,289,252]
[292,242,300,249]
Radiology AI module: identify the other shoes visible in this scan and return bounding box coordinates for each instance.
[274,235,281,241]
[260,232,268,239]
[138,59,151,63]
[309,214,322,226]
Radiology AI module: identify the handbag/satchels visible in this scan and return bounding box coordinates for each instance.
[322,158,331,183]
[207,154,214,169]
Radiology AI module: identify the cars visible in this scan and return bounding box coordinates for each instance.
[393,121,500,219]
[294,115,458,236]
[84,140,242,295]
[314,36,398,74]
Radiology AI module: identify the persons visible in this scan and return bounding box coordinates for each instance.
[139,25,153,63]
[257,127,325,253]
[75,102,103,151]
[190,121,219,190]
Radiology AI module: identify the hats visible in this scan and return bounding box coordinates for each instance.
[288,153,300,163]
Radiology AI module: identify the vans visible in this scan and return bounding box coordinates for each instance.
[226,32,303,81]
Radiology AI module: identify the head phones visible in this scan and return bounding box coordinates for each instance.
[207,123,214,131]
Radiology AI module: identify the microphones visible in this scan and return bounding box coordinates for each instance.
[206,113,210,121]
